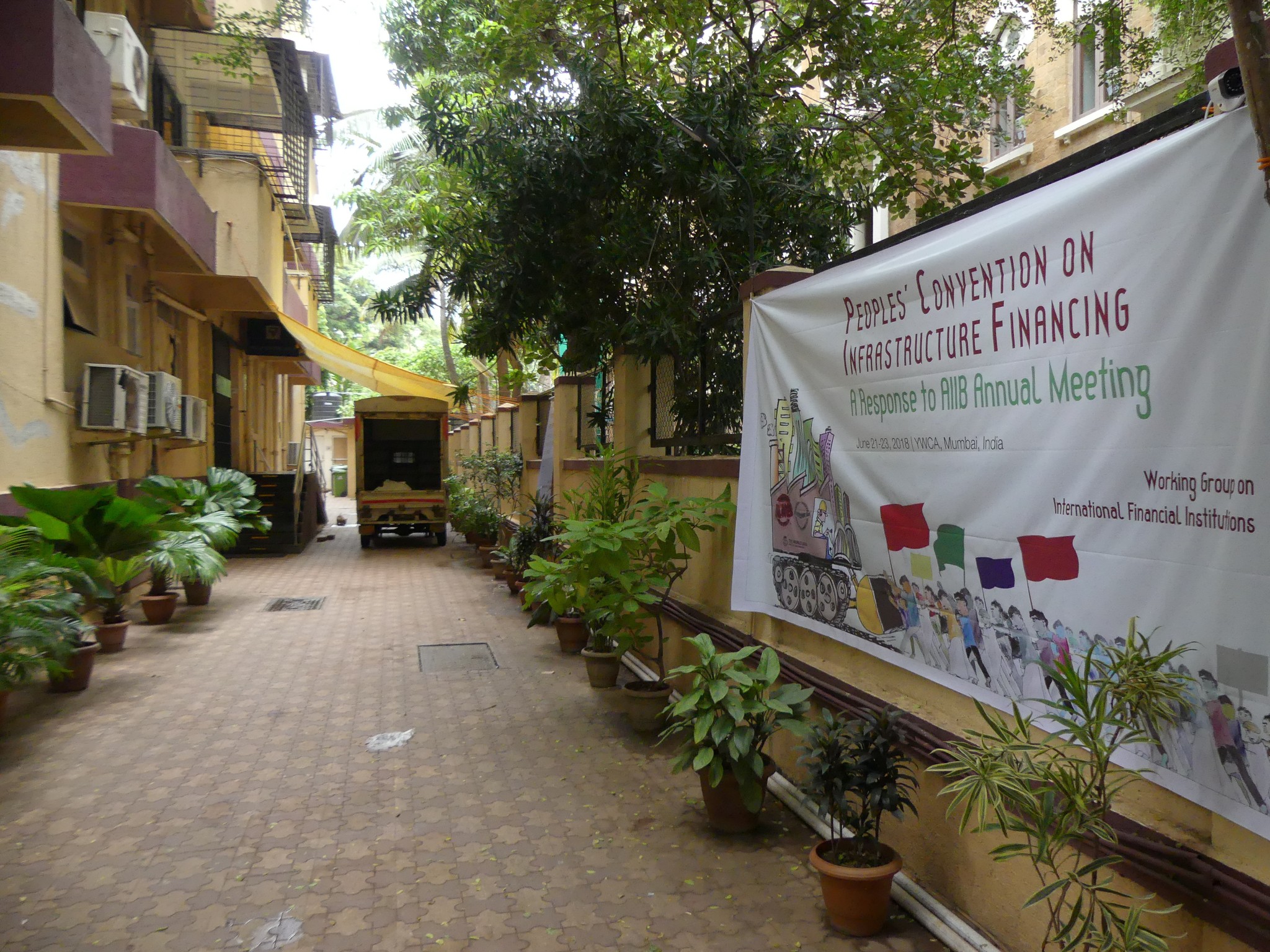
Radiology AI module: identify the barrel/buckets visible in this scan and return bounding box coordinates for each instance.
[313,392,342,422]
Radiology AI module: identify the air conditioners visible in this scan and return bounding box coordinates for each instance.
[170,395,207,441]
[287,442,299,466]
[81,363,150,434]
[140,371,182,431]
[83,10,148,113]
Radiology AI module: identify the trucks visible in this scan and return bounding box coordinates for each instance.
[352,395,448,549]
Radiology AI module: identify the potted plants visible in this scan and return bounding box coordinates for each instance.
[0,466,272,694]
[443,442,921,938]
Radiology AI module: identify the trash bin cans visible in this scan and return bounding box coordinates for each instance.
[330,464,348,495]
[331,469,347,497]
